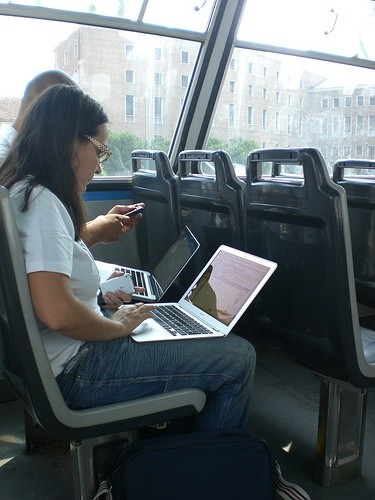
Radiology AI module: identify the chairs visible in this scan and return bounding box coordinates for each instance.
[0,147,375,500]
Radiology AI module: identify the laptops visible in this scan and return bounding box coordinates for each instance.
[94,225,201,303]
[104,244,278,342]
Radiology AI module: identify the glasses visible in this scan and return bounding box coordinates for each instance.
[85,135,112,163]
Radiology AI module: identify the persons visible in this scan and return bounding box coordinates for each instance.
[0,69,146,250]
[0,84,257,432]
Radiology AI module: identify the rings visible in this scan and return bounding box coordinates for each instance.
[135,304,139,308]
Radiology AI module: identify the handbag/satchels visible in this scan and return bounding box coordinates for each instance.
[93,430,311,500]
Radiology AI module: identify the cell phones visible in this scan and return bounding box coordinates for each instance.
[122,205,144,217]
[99,273,135,296]
